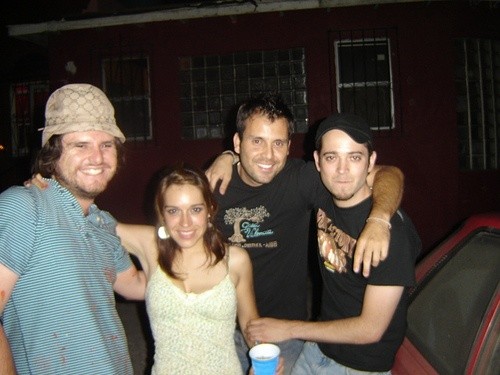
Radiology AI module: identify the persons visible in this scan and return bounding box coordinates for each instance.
[0,83,147,375]
[204,113,423,375]
[208,94,404,375]
[23,168,284,375]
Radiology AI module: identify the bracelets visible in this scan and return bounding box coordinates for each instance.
[222,150,236,165]
[365,216,391,229]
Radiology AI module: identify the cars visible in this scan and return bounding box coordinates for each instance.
[389,209,500,375]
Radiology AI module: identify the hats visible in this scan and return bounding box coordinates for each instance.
[41,84,126,147]
[314,112,370,149]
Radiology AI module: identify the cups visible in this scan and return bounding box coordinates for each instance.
[250,342,279,375]
